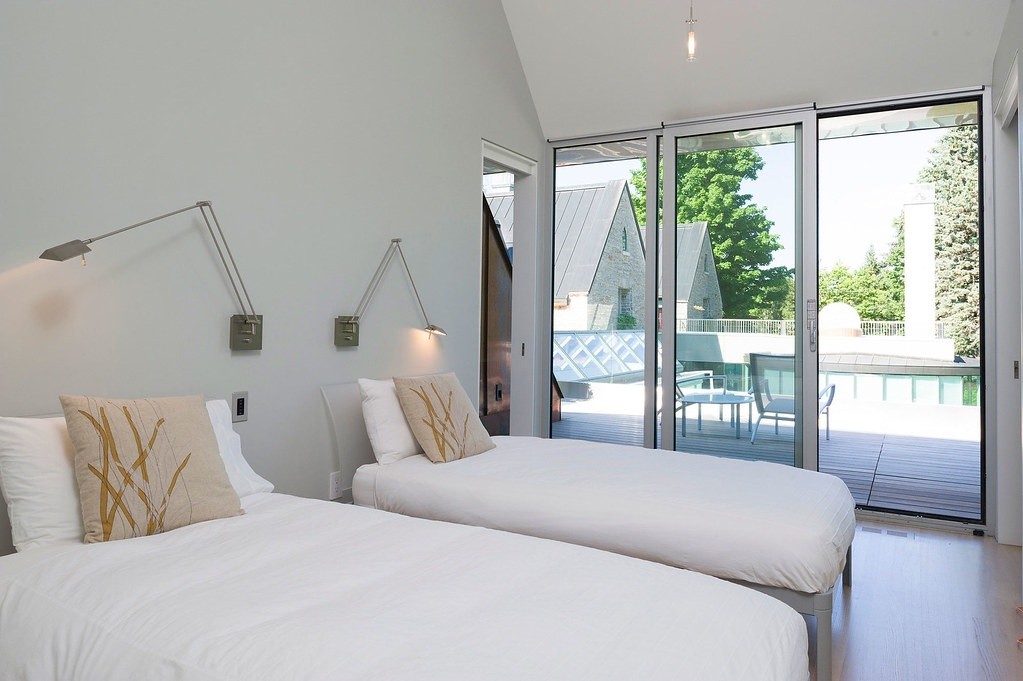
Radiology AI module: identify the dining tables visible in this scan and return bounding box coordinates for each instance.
[678,394,755,439]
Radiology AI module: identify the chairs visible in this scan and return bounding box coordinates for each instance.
[749,352,835,445]
[657,348,727,425]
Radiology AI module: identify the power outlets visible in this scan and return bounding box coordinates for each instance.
[329,471,343,501]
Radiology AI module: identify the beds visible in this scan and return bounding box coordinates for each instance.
[352,435,857,681]
[0,491,811,681]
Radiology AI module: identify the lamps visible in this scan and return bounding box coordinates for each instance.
[39,201,262,350]
[335,238,447,346]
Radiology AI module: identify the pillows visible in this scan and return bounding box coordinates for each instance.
[0,399,275,553]
[357,378,425,466]
[59,394,246,543]
[392,372,496,464]
